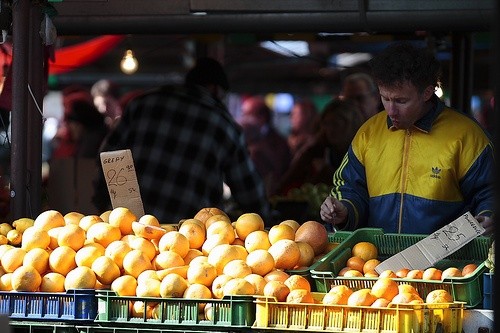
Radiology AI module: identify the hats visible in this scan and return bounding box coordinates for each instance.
[64,101,106,130]
[191,57,231,90]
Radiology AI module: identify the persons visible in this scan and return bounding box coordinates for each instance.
[239,70,384,205]
[41,80,126,216]
[92,58,263,223]
[320,42,498,242]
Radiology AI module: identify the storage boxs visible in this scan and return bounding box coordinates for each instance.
[0,228,500,333]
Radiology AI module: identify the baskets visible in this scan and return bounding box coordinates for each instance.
[75,325,213,333]
[252,296,467,333]
[266,227,353,282]
[310,228,492,308]
[483,272,496,309]
[9,321,75,333]
[95,290,253,328]
[0,289,95,321]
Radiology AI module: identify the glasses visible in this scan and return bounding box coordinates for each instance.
[346,91,373,103]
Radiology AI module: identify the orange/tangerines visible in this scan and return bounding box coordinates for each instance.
[0,208,478,319]
